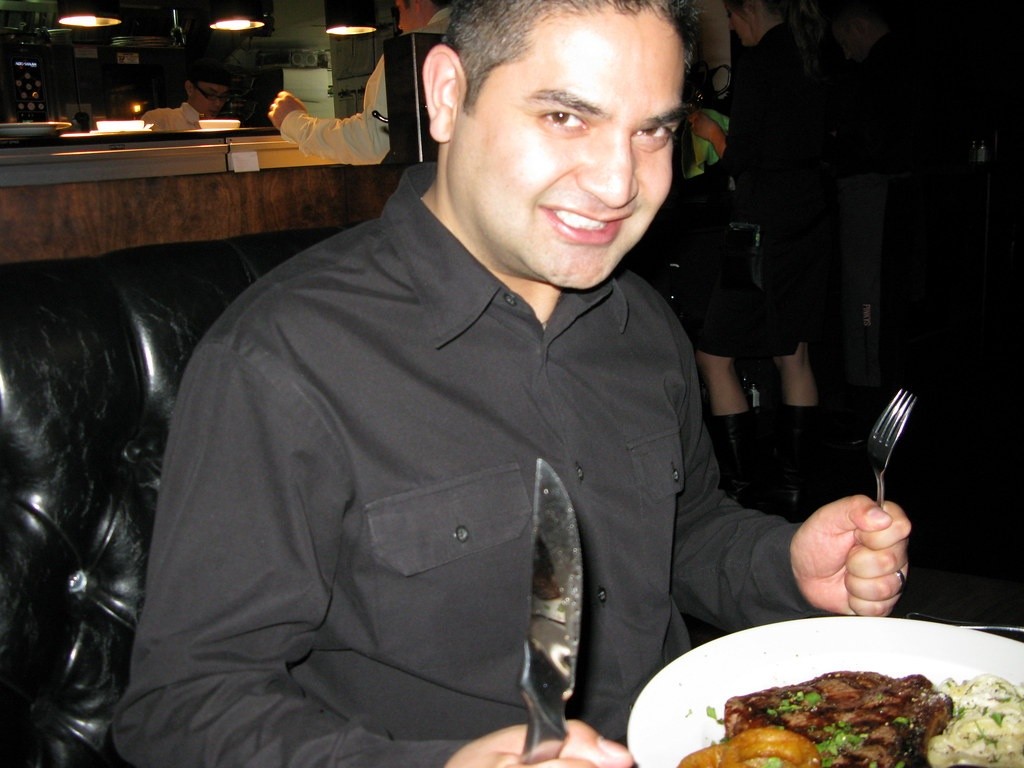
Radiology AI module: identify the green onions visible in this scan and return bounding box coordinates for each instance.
[705,690,909,768]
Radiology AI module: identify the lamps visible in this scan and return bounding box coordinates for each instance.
[206,0,268,32]
[57,0,124,30]
[324,0,379,39]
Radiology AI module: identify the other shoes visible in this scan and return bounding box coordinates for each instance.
[825,409,872,449]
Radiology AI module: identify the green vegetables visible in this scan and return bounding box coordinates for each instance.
[951,695,1013,747]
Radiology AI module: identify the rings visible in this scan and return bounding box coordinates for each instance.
[895,569,907,587]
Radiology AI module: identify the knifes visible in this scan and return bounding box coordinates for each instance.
[517,458,583,768]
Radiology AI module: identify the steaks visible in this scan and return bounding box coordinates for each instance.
[724,671,954,768]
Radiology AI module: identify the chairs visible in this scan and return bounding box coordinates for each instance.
[0,222,373,768]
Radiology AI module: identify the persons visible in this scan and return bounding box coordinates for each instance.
[829,2,931,459]
[110,0,914,768]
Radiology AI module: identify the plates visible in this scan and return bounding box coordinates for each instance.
[628,617,1024,768]
[198,119,241,130]
[94,119,145,133]
[0,122,72,138]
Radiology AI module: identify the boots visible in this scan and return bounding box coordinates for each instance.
[764,404,823,521]
[712,412,762,507]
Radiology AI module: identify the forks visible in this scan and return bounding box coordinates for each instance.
[865,388,917,513]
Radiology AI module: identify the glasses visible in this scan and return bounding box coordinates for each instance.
[191,81,232,102]
